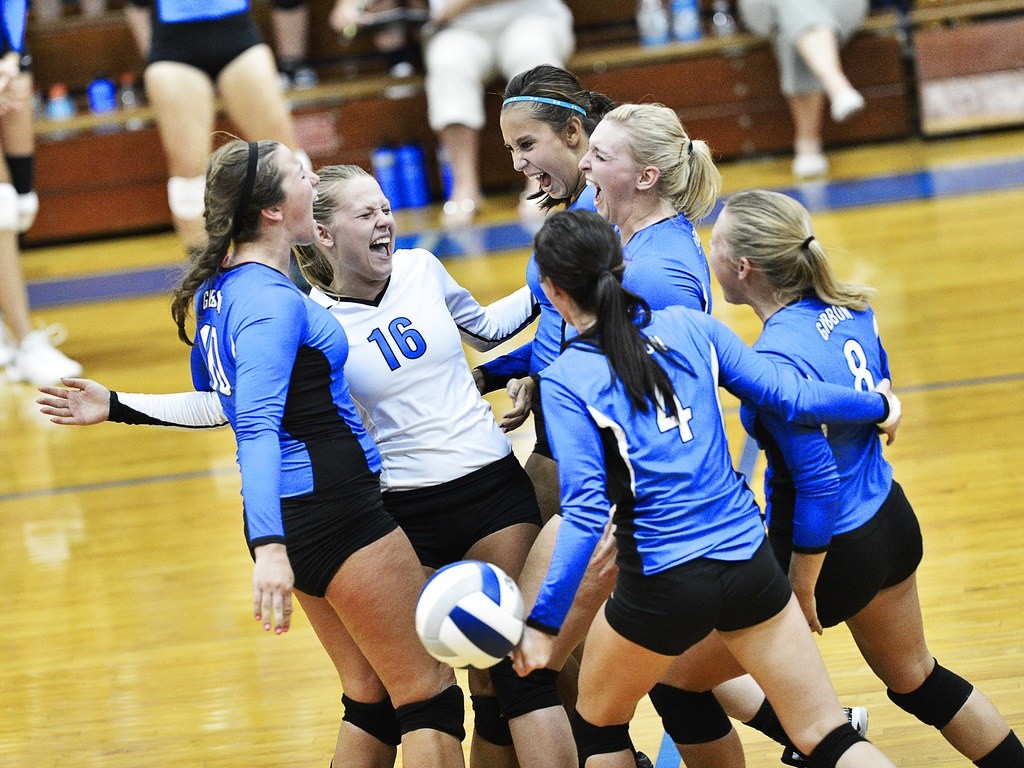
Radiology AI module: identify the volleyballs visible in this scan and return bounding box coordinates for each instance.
[414,557,528,672]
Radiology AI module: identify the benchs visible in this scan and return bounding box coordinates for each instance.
[24,1,1024,245]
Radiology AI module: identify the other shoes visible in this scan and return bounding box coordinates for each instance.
[437,198,484,228]
[831,90,864,122]
[840,706,868,737]
[0,341,14,366]
[516,192,549,215]
[12,324,83,386]
[792,153,829,177]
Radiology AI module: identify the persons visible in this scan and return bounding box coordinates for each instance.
[736,0,868,177]
[421,0,575,227]
[123,0,312,251]
[36,65,1024,768]
[0,0,82,387]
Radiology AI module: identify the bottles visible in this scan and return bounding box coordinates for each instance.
[120,73,146,133]
[20,54,79,141]
[371,145,429,211]
[85,69,120,137]
[637,0,669,44]
[712,0,739,37]
[437,144,454,200]
[672,0,701,40]
